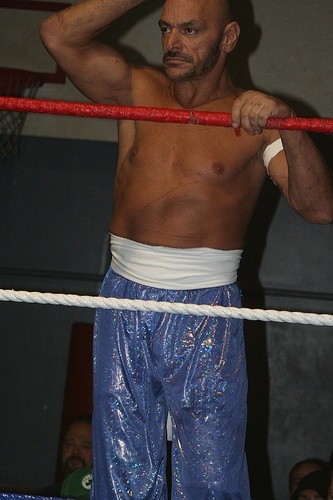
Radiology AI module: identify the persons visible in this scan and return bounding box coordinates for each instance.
[40,0,332,500]
[292,468,329,500]
[58,418,94,472]
[286,458,332,500]
[61,463,91,499]
